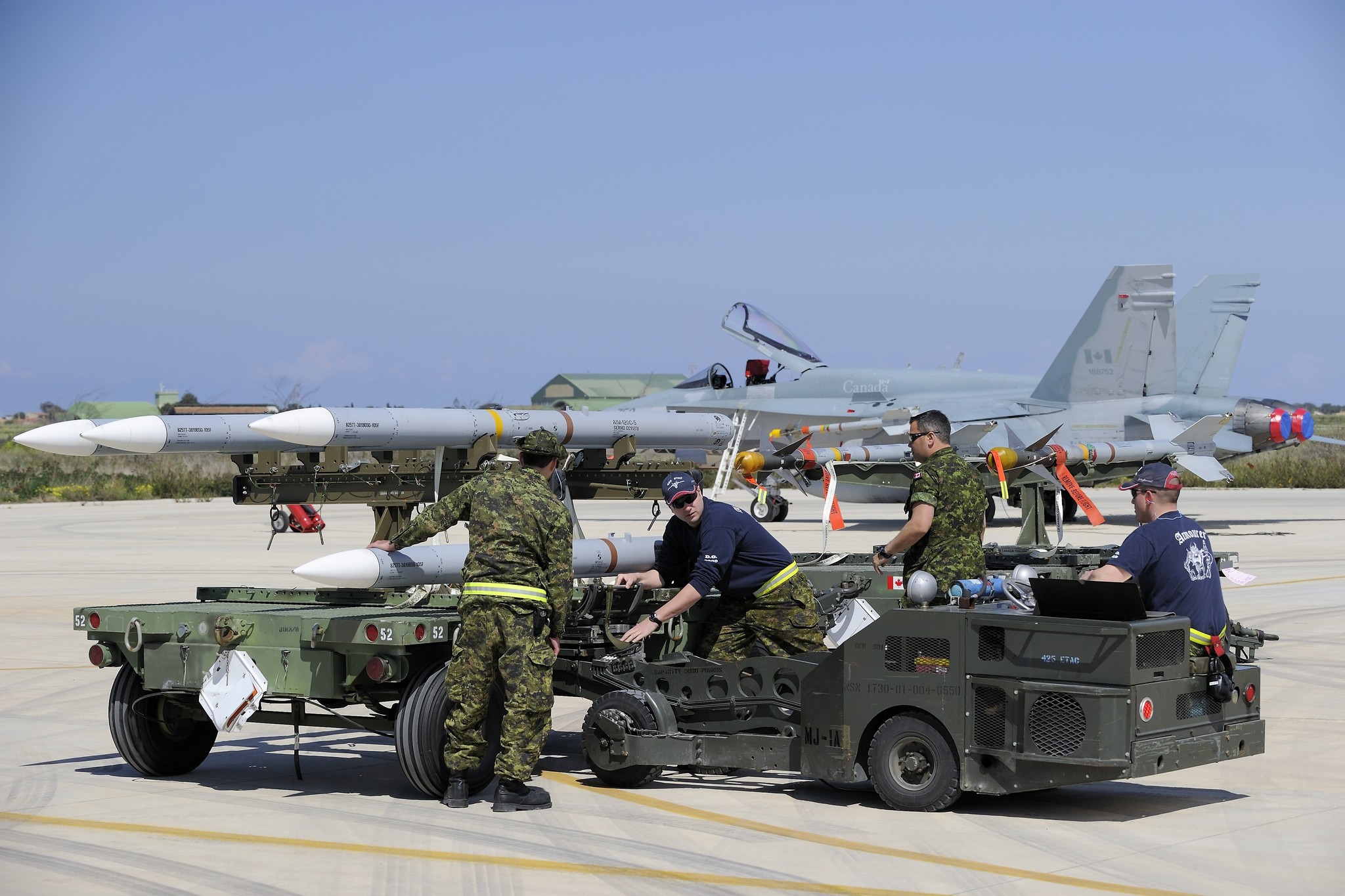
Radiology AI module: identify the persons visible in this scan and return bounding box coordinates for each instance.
[618,470,832,663]
[872,405,989,609]
[1079,462,1229,657]
[366,426,577,812]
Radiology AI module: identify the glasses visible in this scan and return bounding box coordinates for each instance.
[1131,489,1156,498]
[555,459,560,469]
[670,492,697,509]
[909,430,940,443]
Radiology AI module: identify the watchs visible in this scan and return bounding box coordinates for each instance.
[878,546,893,559]
[649,612,663,626]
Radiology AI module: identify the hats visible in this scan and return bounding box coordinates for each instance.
[515,426,568,462]
[1118,462,1183,491]
[661,471,698,506]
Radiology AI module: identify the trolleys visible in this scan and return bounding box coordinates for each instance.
[271,503,326,533]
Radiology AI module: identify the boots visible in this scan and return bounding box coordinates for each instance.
[443,768,470,808]
[493,778,552,812]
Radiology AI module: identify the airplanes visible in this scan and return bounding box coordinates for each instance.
[592,263,1345,525]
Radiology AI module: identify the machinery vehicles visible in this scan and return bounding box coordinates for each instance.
[71,409,1280,812]
